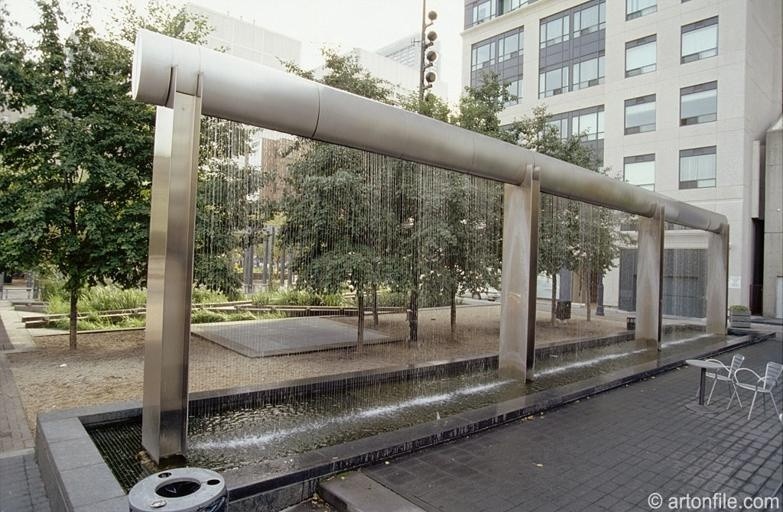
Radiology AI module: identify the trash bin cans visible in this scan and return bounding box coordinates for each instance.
[129,466,228,512]
[556,301,571,322]
[627,317,635,333]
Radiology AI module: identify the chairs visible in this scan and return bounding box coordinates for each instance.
[729,361,783,422]
[696,354,745,408]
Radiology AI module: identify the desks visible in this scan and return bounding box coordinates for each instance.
[685,358,730,406]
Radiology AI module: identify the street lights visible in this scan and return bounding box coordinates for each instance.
[417,0,436,105]
